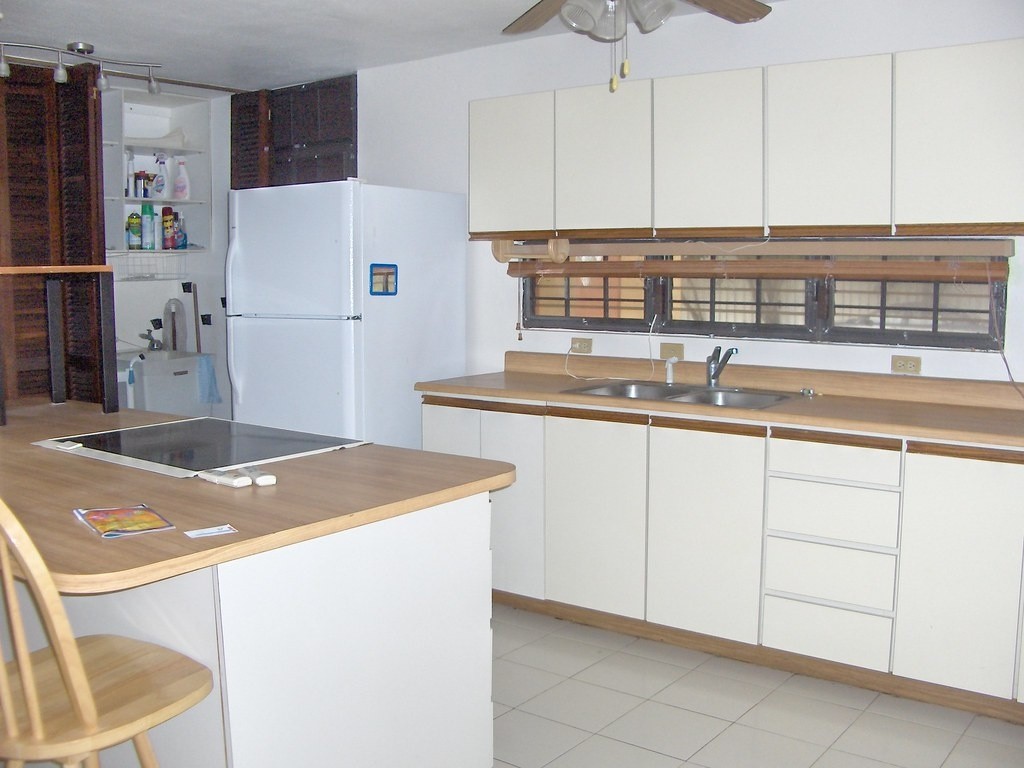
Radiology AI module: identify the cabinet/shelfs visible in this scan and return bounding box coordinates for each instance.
[467,37,1024,237]
[421,398,1024,730]
[105,83,222,417]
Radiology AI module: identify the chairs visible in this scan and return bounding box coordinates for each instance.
[1,500,214,768]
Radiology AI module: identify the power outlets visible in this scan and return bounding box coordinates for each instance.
[571,338,592,353]
[892,356,921,372]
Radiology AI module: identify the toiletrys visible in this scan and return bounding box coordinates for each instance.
[124,150,191,196]
[125,203,189,251]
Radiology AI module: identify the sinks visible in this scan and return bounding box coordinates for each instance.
[667,390,788,409]
[572,382,696,399]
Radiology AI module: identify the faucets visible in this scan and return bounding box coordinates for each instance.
[706,345,738,388]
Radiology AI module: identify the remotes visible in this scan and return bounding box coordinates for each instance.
[197,470,252,488]
[239,467,277,487]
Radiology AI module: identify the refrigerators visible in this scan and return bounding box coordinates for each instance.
[226,180,468,452]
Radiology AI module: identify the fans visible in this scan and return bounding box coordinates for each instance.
[503,0,773,37]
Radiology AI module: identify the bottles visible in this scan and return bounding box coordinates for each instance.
[174,210,188,249]
[136,170,149,198]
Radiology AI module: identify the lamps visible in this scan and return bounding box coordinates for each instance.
[563,0,676,91]
[0,41,166,95]
